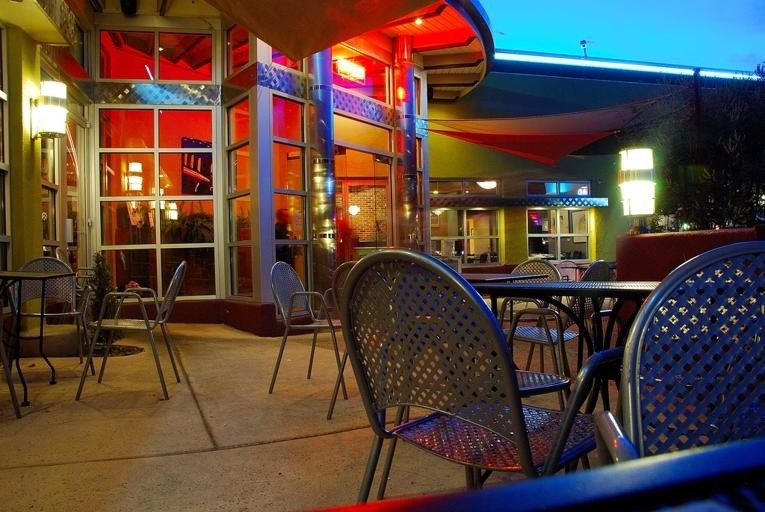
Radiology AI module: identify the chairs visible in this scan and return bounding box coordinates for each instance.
[327,260,361,418]
[269,261,351,402]
[504,260,614,370]
[56,247,96,324]
[500,259,563,339]
[0,341,22,418]
[586,242,765,464]
[6,256,96,376]
[75,260,188,400]
[331,246,598,505]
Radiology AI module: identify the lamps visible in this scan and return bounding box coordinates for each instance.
[30,80,69,140]
[618,146,657,216]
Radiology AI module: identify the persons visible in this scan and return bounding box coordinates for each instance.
[275,208,301,271]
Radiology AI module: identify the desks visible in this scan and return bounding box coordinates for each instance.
[463,258,592,274]
[454,270,550,286]
[0,270,75,406]
[468,276,667,471]
[428,248,520,273]
